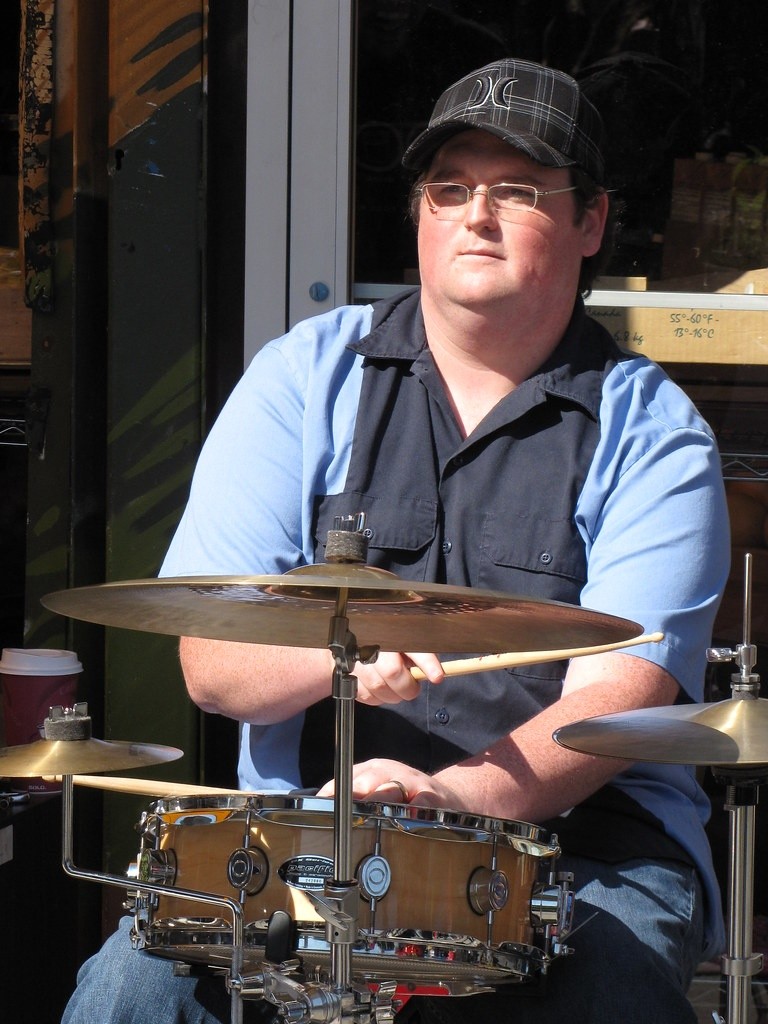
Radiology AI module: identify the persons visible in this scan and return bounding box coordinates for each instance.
[58,60,731,1024]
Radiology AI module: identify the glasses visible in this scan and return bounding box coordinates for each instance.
[417,180,585,221]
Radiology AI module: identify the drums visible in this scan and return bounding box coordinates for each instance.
[131,793,564,979]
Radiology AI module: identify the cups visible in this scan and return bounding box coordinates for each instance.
[0,648,83,748]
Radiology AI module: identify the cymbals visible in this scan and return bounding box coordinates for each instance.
[553,694,767,765]
[39,557,643,654]
[1,737,185,778]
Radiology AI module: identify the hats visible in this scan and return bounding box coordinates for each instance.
[405,59,611,199]
